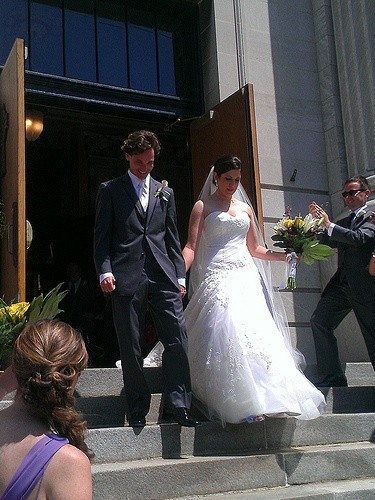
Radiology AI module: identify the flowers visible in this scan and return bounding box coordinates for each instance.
[155,179,171,202]
[270,204,337,292]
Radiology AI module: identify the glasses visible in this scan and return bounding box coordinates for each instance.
[342,189,364,197]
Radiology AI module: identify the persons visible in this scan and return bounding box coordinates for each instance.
[0,318,94,500]
[57,263,120,368]
[93,130,327,427]
[308,175,375,444]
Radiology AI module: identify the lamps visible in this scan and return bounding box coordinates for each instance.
[25,108,46,141]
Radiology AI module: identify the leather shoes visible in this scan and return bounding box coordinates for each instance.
[162,404,201,427]
[313,374,347,387]
[128,414,145,427]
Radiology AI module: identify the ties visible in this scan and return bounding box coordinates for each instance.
[69,282,75,295]
[347,213,355,228]
[138,181,149,213]
